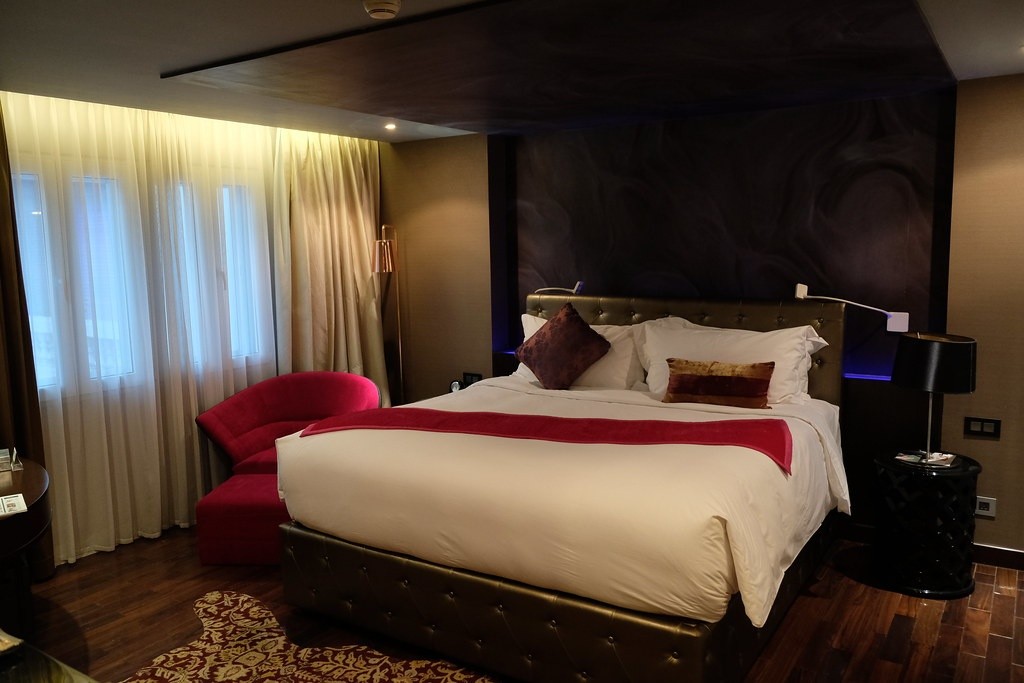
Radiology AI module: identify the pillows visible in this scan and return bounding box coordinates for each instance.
[515,302,611,389]
[629,317,829,404]
[508,314,644,390]
[661,358,775,410]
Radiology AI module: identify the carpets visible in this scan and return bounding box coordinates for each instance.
[113,591,493,683]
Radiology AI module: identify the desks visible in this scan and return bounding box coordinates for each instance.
[0,459,53,626]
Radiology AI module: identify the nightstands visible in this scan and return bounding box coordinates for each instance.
[885,452,982,599]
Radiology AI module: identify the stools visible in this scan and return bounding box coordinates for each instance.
[195,474,289,568]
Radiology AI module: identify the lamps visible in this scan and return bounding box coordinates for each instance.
[894,332,976,466]
[370,223,404,406]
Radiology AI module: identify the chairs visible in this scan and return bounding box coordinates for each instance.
[194,372,382,472]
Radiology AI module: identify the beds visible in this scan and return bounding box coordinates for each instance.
[278,299,846,683]
